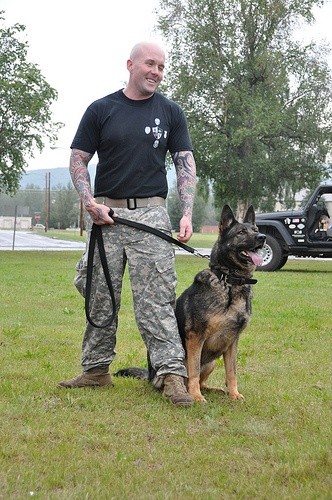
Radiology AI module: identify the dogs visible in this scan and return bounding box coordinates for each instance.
[113,203,266,402]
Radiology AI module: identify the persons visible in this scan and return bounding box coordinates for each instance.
[58,42,197,407]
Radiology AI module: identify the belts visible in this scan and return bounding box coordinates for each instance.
[93,196,167,209]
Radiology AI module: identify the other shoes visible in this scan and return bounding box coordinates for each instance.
[161,374,193,407]
[55,366,115,391]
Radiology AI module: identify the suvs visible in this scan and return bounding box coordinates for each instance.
[253,182,332,273]
